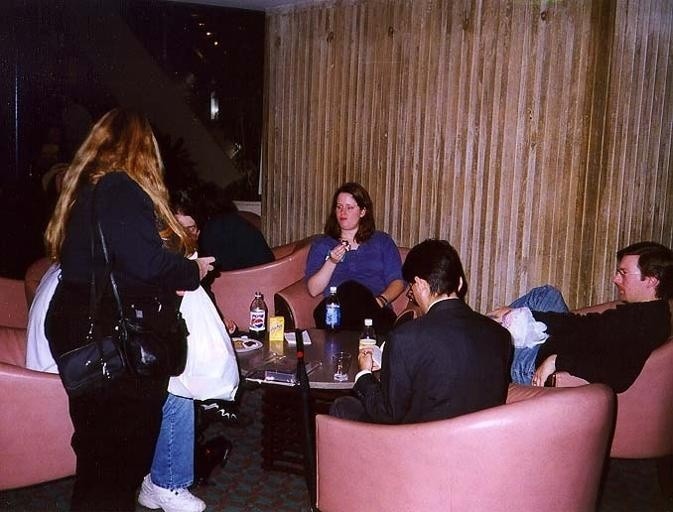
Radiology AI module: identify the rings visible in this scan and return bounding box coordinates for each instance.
[533,381,536,384]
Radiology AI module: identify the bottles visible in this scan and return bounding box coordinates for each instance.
[358,319,376,358]
[323,334,339,370]
[248,291,267,338]
[322,286,342,331]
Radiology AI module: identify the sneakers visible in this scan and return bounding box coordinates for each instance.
[138,473,206,512]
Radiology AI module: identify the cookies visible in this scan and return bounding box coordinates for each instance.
[340,239,349,247]
[234,340,244,350]
[244,341,258,348]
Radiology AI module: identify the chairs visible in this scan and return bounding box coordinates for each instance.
[1,209,673,512]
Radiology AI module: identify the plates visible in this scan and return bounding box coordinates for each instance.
[230,335,262,353]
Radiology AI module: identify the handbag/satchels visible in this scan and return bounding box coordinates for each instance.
[118,305,187,378]
[56,319,125,397]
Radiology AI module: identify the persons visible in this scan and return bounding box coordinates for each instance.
[330,238,514,424]
[172,207,220,293]
[25,258,207,512]
[305,182,407,331]
[482,241,673,396]
[197,182,276,269]
[43,109,214,512]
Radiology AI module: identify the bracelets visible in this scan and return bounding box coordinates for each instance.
[377,295,388,307]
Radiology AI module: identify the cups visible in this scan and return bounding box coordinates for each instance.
[331,350,352,381]
